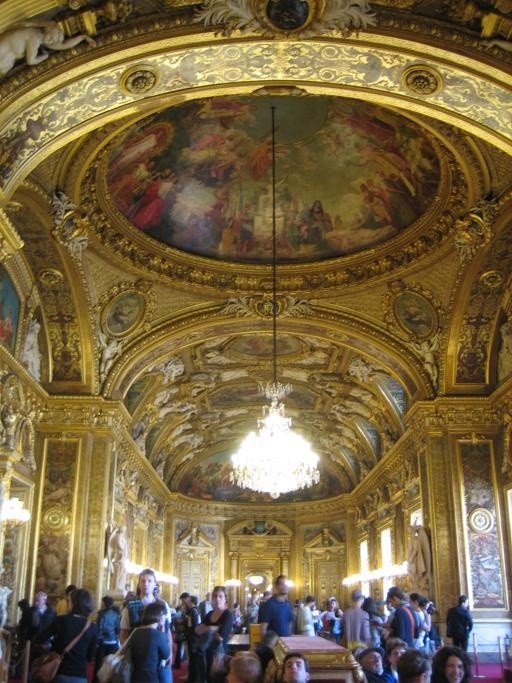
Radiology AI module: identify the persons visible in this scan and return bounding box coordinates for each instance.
[481,38,512,53]
[406,524,431,586]
[412,327,443,388]
[0,27,99,76]
[106,526,128,569]
[16,566,475,683]
[96,333,123,382]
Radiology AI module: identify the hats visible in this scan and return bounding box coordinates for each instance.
[353,646,385,660]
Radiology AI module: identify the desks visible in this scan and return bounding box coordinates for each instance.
[227,635,250,656]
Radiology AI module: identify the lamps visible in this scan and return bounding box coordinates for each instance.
[228,107,322,500]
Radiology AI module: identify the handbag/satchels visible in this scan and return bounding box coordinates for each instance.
[95,653,126,683]
[31,650,62,683]
[429,628,441,647]
